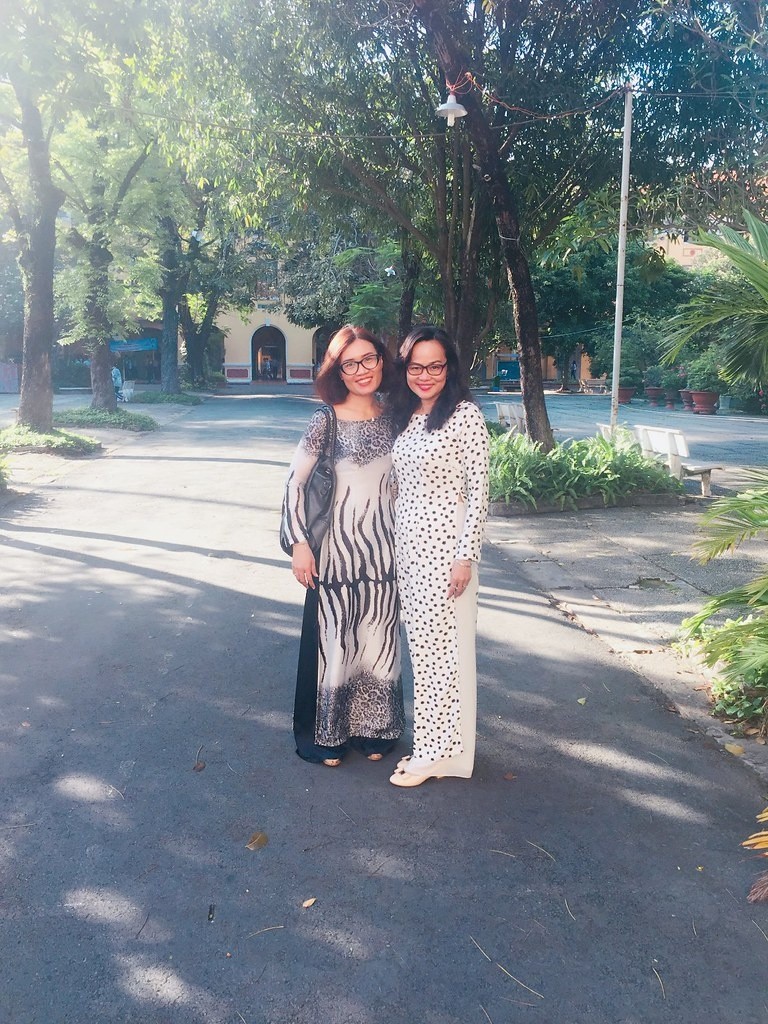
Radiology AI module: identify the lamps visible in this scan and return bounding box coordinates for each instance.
[436,92,468,126]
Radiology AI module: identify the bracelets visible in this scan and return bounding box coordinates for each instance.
[291,542,309,547]
[454,560,473,570]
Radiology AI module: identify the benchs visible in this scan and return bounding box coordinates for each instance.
[494,402,514,427]
[508,403,526,432]
[634,426,723,497]
[117,381,134,402]
[597,423,635,448]
[587,379,606,395]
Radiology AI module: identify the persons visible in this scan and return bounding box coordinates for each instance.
[110,362,125,405]
[378,324,493,788]
[264,357,275,381]
[277,325,404,766]
[570,360,578,380]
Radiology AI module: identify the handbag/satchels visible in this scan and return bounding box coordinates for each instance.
[280,405,337,558]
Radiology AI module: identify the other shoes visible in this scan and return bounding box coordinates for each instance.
[121,396,125,402]
[323,759,341,766]
[368,753,383,760]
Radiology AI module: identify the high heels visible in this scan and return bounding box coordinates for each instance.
[389,754,445,787]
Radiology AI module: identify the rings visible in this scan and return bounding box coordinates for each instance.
[295,573,300,578]
[463,585,468,588]
[450,583,458,590]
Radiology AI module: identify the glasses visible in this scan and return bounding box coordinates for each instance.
[340,354,380,375]
[407,361,447,376]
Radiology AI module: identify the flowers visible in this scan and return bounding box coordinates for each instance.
[757,385,768,416]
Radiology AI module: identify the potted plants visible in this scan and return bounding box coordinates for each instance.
[688,348,720,415]
[644,365,664,407]
[679,373,693,412]
[663,369,679,409]
[605,367,642,403]
